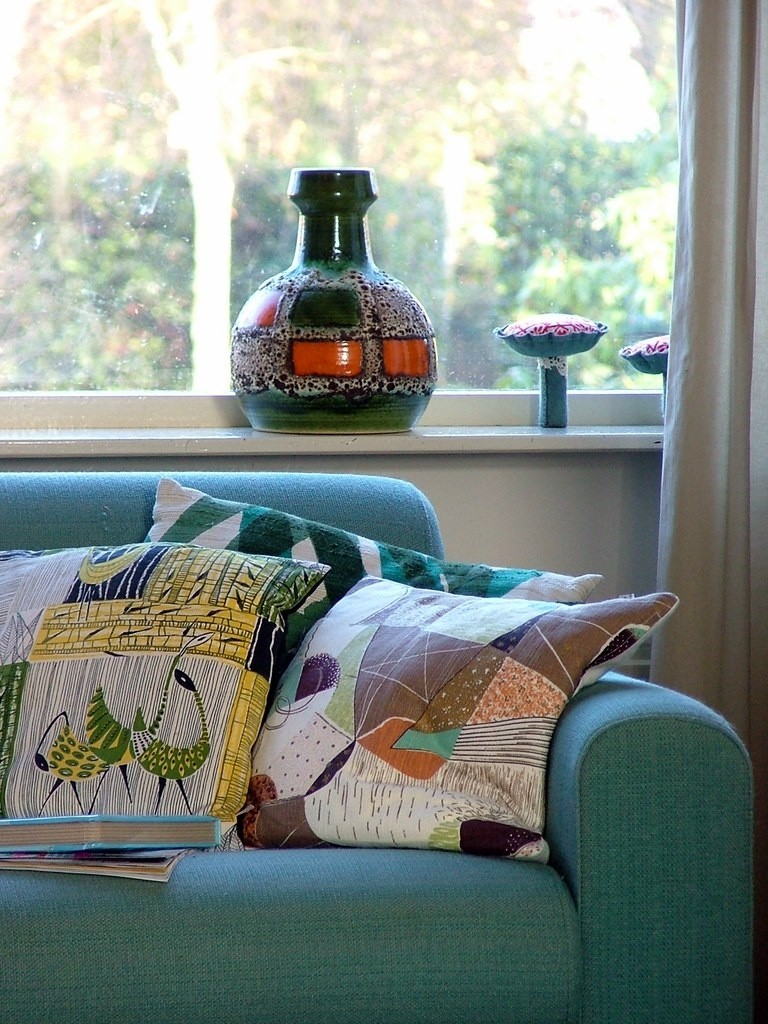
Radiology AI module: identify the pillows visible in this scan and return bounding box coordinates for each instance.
[0,543,332,854]
[143,478,604,610]
[236,572,682,864]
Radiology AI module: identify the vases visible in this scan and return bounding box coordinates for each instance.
[230,168,439,435]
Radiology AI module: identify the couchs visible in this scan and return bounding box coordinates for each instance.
[0,471,760,1024]
